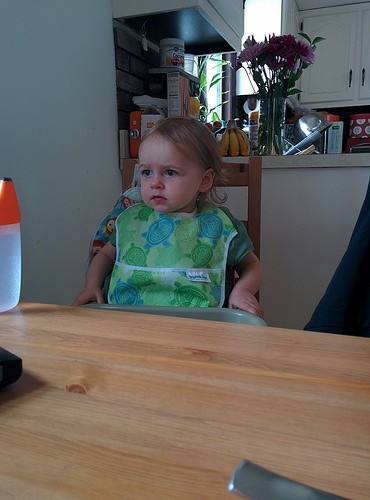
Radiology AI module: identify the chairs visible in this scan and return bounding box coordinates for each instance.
[78,156,267,326]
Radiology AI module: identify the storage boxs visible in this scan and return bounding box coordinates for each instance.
[350,113,370,138]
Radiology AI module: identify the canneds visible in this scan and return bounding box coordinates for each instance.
[160,38,185,69]
[188,96,199,121]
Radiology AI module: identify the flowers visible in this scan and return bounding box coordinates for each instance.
[234,33,326,97]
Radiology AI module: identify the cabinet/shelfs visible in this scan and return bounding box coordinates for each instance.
[295,1,370,109]
[112,0,246,56]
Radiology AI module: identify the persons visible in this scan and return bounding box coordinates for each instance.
[70,115,264,321]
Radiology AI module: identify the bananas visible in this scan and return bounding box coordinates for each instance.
[219,119,251,158]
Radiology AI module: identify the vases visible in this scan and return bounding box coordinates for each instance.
[259,97,286,156]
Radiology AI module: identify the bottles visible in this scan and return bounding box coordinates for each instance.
[0,176,22,313]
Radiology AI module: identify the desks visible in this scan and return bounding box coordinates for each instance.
[0,302,370,500]
